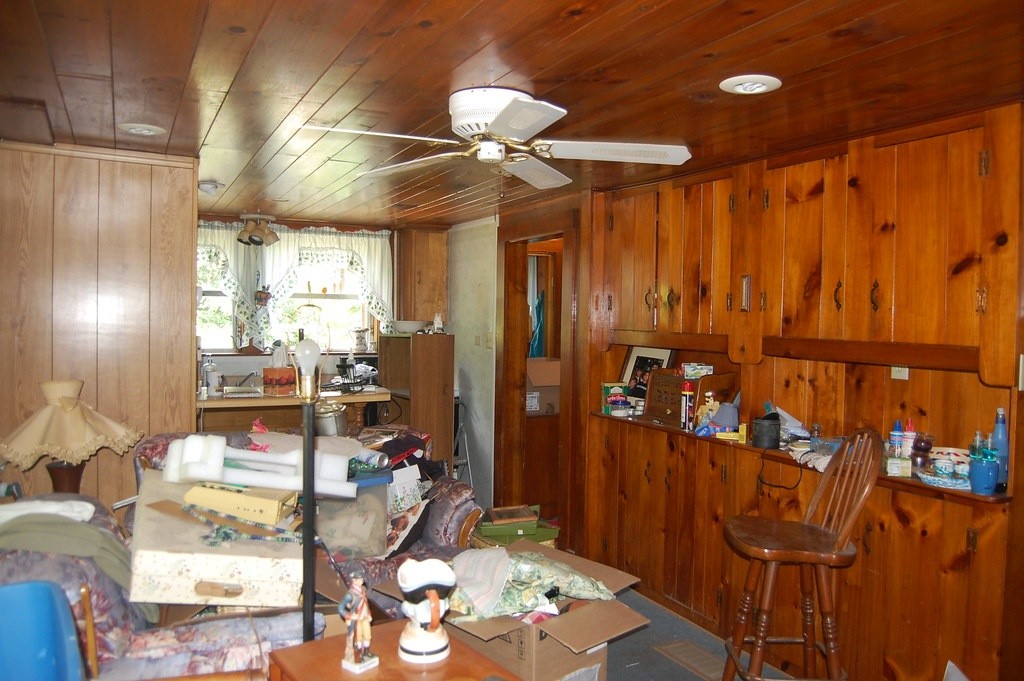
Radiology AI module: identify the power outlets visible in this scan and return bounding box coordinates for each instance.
[474,336,481,347]
[890,367,910,381]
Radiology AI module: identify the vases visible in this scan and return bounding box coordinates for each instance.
[352,326,370,353]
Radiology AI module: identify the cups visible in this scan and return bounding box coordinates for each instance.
[913,432,935,452]
[911,451,931,470]
[206,371,224,396]
[752,416,780,449]
[609,400,631,416]
[969,460,999,495]
[934,460,955,477]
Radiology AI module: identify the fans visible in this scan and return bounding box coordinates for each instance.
[296,86,693,191]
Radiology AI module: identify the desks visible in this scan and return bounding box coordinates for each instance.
[196,382,391,430]
[266,617,527,681]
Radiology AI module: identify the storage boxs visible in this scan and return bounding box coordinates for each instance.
[600,382,628,414]
[682,362,714,381]
[387,464,423,514]
[527,357,561,418]
[370,502,649,681]
[128,465,306,609]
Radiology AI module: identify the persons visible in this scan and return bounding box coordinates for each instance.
[627,361,661,399]
[338,571,374,664]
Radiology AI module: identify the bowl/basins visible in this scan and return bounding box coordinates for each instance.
[390,320,428,333]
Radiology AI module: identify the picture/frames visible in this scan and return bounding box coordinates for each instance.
[618,346,678,408]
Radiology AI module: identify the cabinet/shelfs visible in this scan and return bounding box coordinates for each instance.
[583,99,1024,681]
[378,334,456,480]
[399,228,450,326]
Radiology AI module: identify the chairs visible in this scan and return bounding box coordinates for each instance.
[336,363,365,396]
[722,427,884,681]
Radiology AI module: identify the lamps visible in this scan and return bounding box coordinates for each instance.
[235,213,280,246]
[286,303,331,642]
[0,378,146,496]
[256,285,272,312]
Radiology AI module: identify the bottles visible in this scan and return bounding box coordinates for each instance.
[889,420,903,458]
[354,403,366,426]
[901,419,916,458]
[991,407,1008,494]
[983,433,997,460]
[788,440,811,451]
[353,447,389,468]
[968,431,983,459]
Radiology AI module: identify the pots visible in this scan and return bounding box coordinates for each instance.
[315,406,348,437]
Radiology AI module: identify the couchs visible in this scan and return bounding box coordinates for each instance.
[0,426,487,681]
